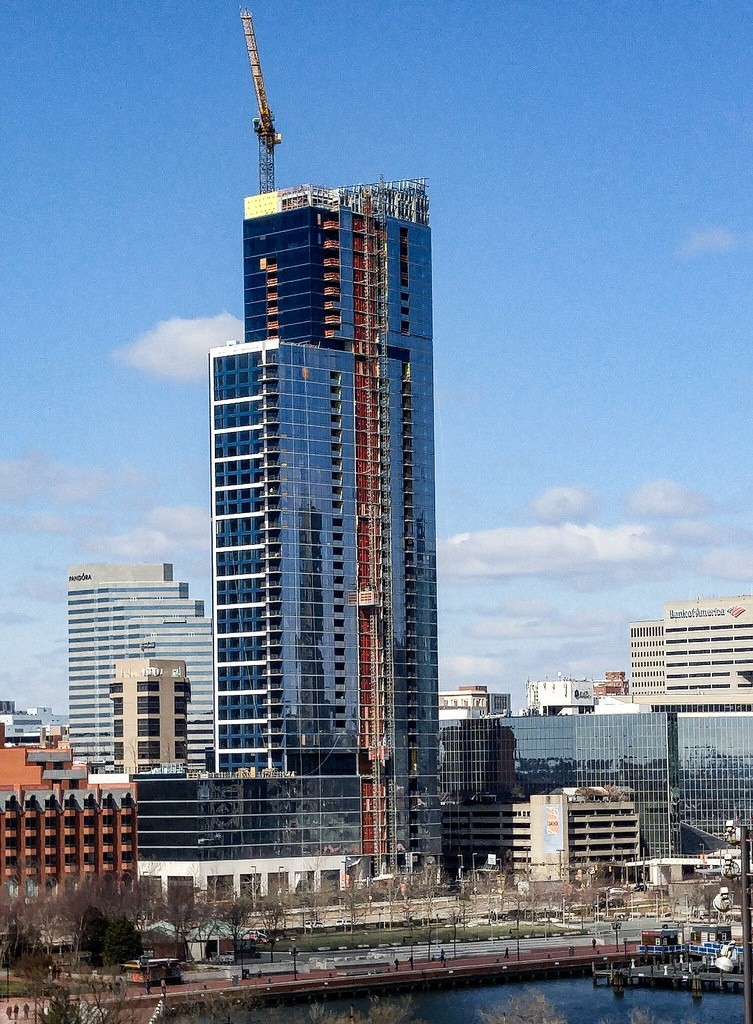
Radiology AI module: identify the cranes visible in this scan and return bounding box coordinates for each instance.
[233,6,280,194]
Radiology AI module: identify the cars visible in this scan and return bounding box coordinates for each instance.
[634,885,647,892]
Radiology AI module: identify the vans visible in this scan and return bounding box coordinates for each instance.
[337,919,352,925]
[305,921,324,927]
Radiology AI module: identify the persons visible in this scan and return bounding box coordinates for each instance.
[161,978,167,993]
[592,938,596,949]
[7,1005,12,1019]
[408,956,412,965]
[24,1004,29,1019]
[504,947,509,958]
[14,1004,19,1020]
[146,979,152,995]
[394,958,399,970]
[441,949,445,962]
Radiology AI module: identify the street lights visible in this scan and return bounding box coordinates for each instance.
[251,866,256,901]
[700,844,705,882]
[596,893,600,921]
[411,851,421,887]
[472,853,478,892]
[557,849,565,879]
[278,867,284,891]
[457,854,463,883]
[629,890,637,922]
[685,893,689,924]
[655,891,658,923]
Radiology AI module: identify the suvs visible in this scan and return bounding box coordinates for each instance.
[609,888,628,895]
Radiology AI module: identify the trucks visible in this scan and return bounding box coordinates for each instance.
[244,931,267,940]
